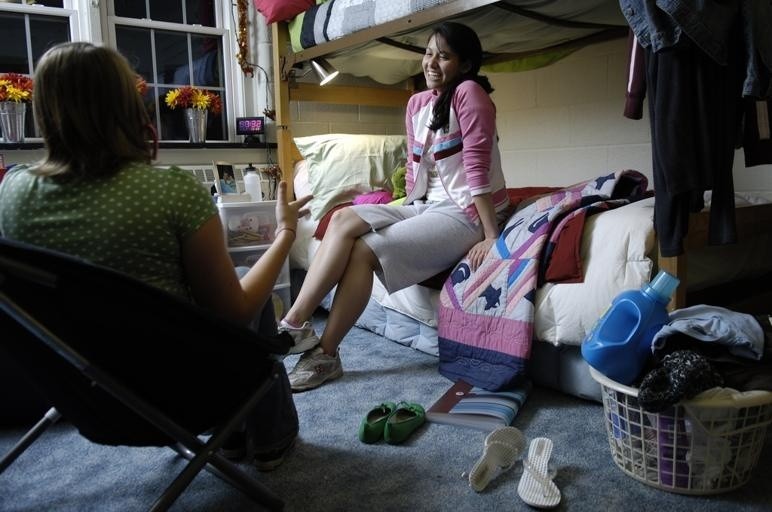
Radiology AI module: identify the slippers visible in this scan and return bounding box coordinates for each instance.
[517,437,561,508]
[469,426,526,489]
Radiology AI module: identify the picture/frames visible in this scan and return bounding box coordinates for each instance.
[215,161,240,196]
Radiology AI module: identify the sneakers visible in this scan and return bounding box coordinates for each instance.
[384,404,424,444]
[288,347,342,392]
[276,320,319,361]
[359,402,395,442]
[217,431,247,461]
[253,439,294,470]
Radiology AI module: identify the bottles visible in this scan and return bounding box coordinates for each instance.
[243,163,262,202]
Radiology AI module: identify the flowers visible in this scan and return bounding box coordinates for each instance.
[0,71,34,100]
[164,85,220,110]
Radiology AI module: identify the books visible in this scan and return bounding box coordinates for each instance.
[423,374,533,427]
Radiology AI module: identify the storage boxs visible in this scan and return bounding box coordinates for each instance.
[211,189,292,319]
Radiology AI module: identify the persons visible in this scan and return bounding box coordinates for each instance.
[219,171,233,186]
[275,21,509,390]
[0,41,313,470]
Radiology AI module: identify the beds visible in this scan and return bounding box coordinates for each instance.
[268,0,772,407]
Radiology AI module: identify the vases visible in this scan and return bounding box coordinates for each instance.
[0,101,25,142]
[185,106,208,142]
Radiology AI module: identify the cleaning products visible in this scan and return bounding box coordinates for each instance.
[581,269,680,385]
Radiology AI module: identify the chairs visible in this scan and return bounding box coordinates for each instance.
[0,236,283,512]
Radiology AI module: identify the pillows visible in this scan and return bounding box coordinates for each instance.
[288,127,406,216]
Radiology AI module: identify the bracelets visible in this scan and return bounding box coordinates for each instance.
[274,228,296,237]
